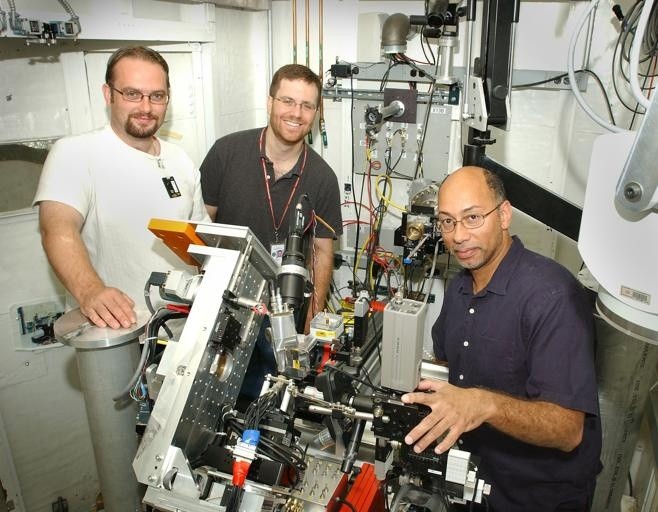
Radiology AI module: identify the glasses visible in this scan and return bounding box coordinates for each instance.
[273,95,319,113]
[111,86,170,106]
[437,199,503,234]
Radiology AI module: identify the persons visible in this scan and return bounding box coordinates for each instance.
[400,166,605,512]
[29,43,214,344]
[198,64,344,414]
